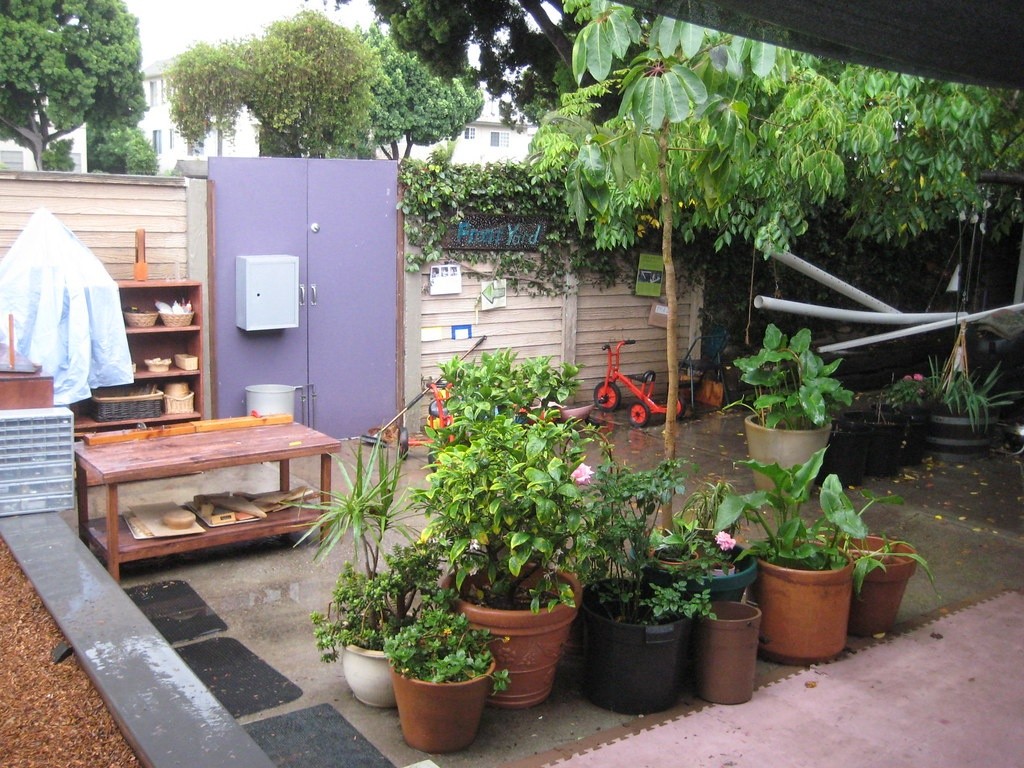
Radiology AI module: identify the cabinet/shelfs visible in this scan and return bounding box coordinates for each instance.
[70,280,204,437]
[0,406,75,519]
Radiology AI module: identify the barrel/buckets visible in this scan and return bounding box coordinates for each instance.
[245,384,296,420]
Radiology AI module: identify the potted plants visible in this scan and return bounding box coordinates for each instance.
[273,353,938,754]
[924,354,1024,463]
[720,325,855,503]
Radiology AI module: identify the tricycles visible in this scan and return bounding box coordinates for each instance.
[397,382,472,473]
[593,340,687,426]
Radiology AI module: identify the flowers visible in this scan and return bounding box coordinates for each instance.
[567,461,595,488]
[883,373,938,414]
[710,531,736,564]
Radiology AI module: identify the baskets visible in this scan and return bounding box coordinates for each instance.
[123,311,158,328]
[159,310,194,327]
[144,359,172,372]
[162,390,194,414]
[88,385,164,422]
[175,354,198,370]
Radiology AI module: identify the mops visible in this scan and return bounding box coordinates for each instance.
[359,334,487,448]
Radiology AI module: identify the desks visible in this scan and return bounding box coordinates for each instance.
[0,362,55,410]
[74,413,341,584]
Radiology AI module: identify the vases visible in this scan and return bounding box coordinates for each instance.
[690,600,762,705]
[869,403,932,467]
[814,419,869,489]
[840,410,907,477]
[706,564,735,577]
